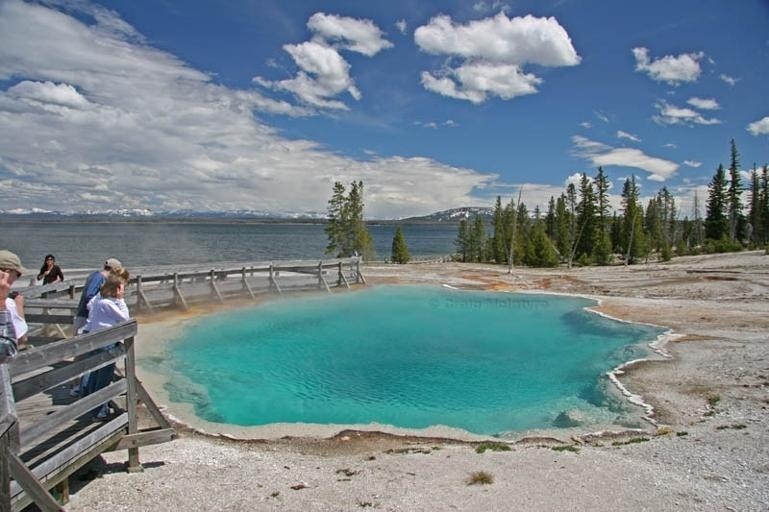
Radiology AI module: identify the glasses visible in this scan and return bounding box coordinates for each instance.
[15,272,22,278]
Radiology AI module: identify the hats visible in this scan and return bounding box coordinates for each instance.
[1,250,28,274]
[104,258,122,269]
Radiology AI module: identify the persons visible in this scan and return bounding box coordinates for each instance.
[79,265,130,400]
[68,256,123,398]
[36,253,64,317]
[0,271,19,365]
[0,249,29,347]
[82,274,130,423]
